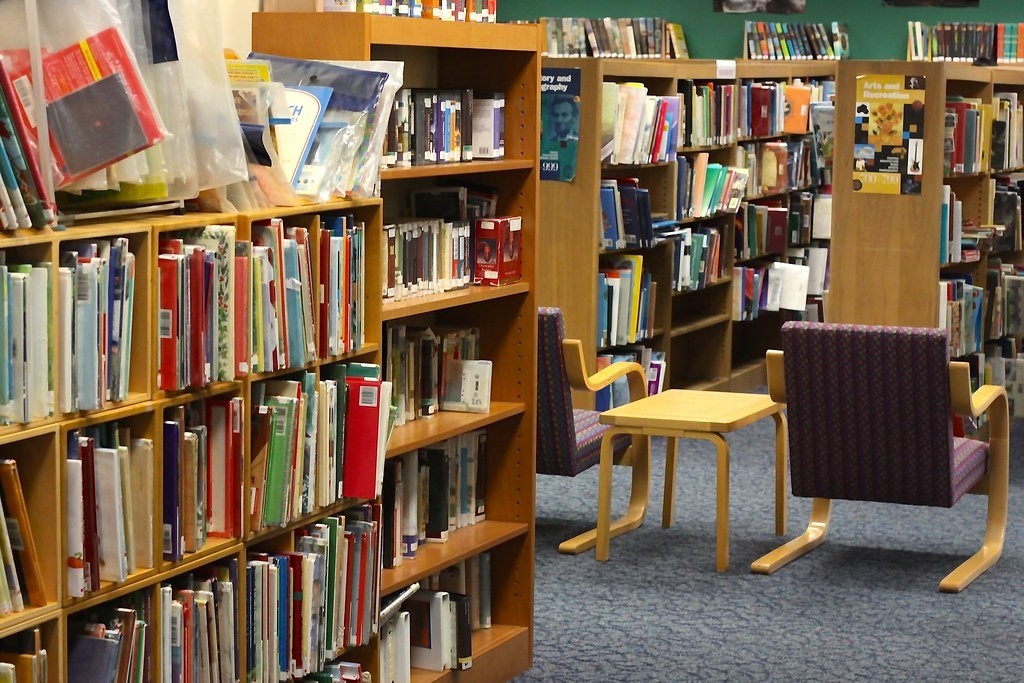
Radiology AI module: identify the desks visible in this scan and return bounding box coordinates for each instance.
[597,389,790,572]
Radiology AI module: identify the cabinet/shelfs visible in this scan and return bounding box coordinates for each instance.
[540,53,837,413]
[251,9,542,683]
[0,198,385,683]
[823,60,1024,442]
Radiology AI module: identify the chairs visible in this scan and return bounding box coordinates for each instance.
[749,319,1012,593]
[535,306,652,556]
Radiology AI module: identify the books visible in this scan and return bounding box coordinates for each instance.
[676,74,837,323]
[322,0,497,24]
[941,176,1024,265]
[0,501,381,683]
[0,216,367,428]
[381,428,488,569]
[596,344,666,415]
[0,0,404,232]
[602,77,677,164]
[377,85,508,168]
[510,11,689,59]
[946,90,1024,174]
[746,19,850,60]
[937,258,1024,359]
[378,549,492,683]
[0,357,393,618]
[907,18,1024,65]
[597,254,658,346]
[382,186,524,301]
[381,319,493,433]
[946,335,1024,438]
[601,175,685,249]
